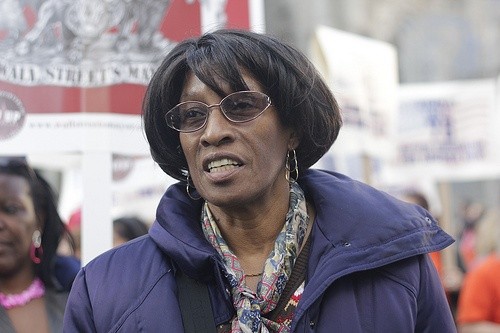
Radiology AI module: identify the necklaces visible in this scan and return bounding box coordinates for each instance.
[242,272,262,279]
[1,277,46,309]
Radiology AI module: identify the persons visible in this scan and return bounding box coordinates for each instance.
[101,183,500,333]
[1,154,79,333]
[61,27,458,333]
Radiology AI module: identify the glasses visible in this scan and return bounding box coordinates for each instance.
[164,90,276,134]
[0,155,37,181]
